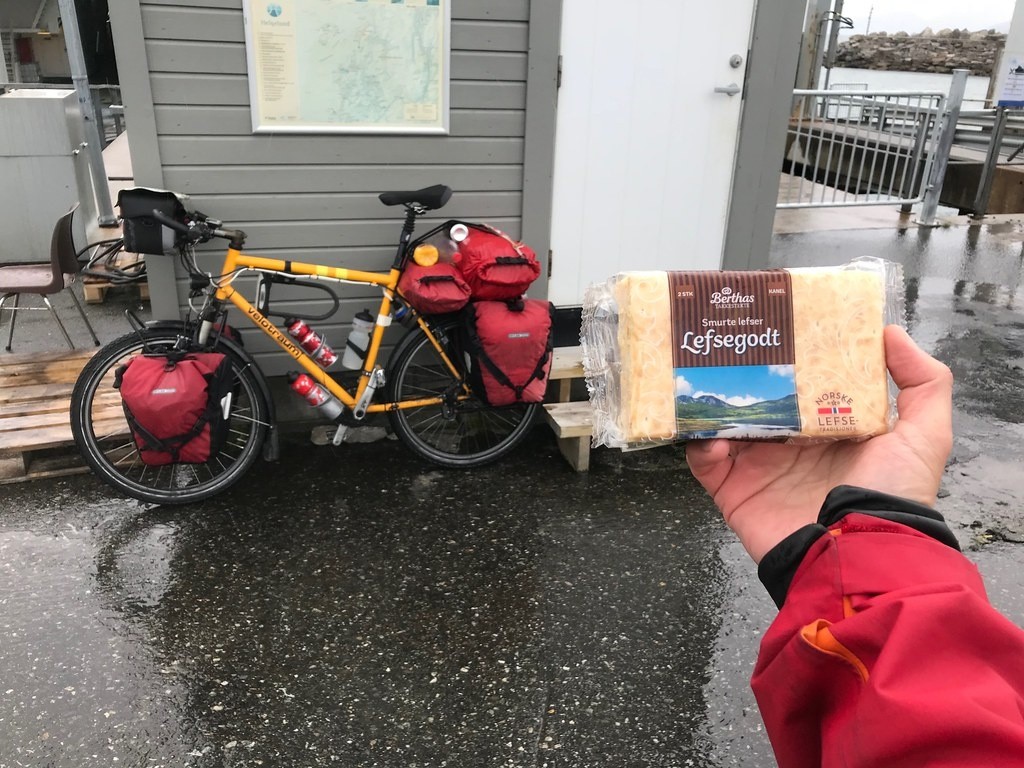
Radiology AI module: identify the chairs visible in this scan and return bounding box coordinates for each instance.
[0,200,101,353]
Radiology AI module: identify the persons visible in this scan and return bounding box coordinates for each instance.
[685,320,1024,768]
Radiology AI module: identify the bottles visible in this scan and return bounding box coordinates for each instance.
[342,308,375,370]
[283,315,339,371]
[286,370,345,422]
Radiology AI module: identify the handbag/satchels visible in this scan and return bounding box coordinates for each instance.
[113,323,244,466]
[398,226,554,406]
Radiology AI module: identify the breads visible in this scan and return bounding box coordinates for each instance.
[618,264,889,438]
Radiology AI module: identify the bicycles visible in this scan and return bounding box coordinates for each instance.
[68,184,538,506]
